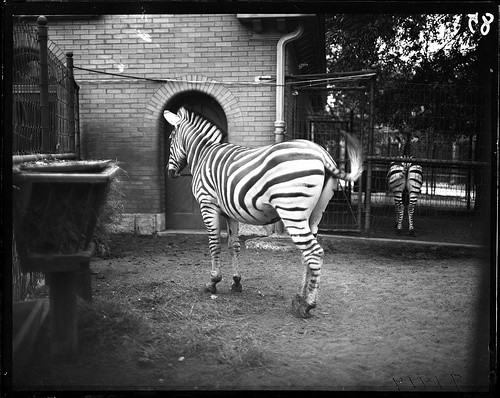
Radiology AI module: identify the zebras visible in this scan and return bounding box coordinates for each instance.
[163,107,365,318]
[387,156,423,235]
